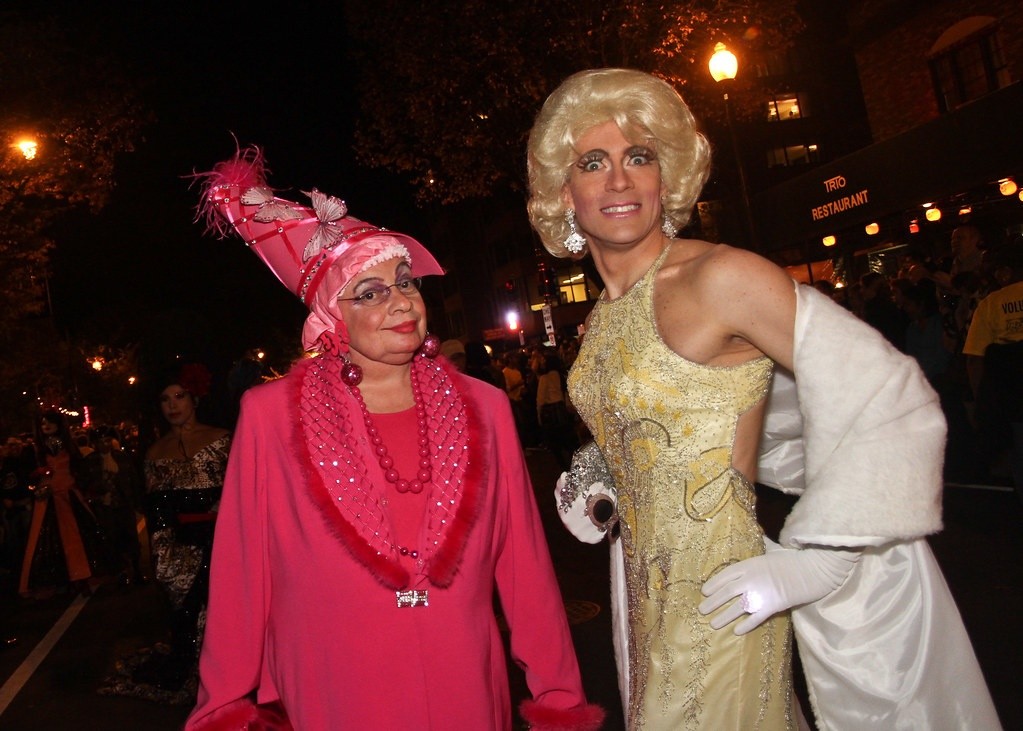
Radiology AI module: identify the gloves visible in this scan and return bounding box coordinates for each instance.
[553,472,619,545]
[697,535,866,637]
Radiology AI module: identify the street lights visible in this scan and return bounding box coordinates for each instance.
[11,134,77,410]
[707,43,764,254]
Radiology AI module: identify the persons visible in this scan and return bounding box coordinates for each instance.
[18,410,93,598]
[0,411,138,576]
[527,69,1002,731]
[961,244,1023,496]
[812,225,1023,402]
[185,235,605,731]
[441,339,578,447]
[97,362,234,705]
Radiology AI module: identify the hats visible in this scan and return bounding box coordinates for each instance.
[185,132,449,353]
[89,424,120,440]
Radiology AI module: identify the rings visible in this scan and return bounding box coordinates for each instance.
[742,592,762,613]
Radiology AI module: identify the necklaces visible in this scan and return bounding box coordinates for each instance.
[352,363,431,493]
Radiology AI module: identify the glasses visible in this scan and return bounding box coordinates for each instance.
[337,276,422,307]
[584,492,621,543]
